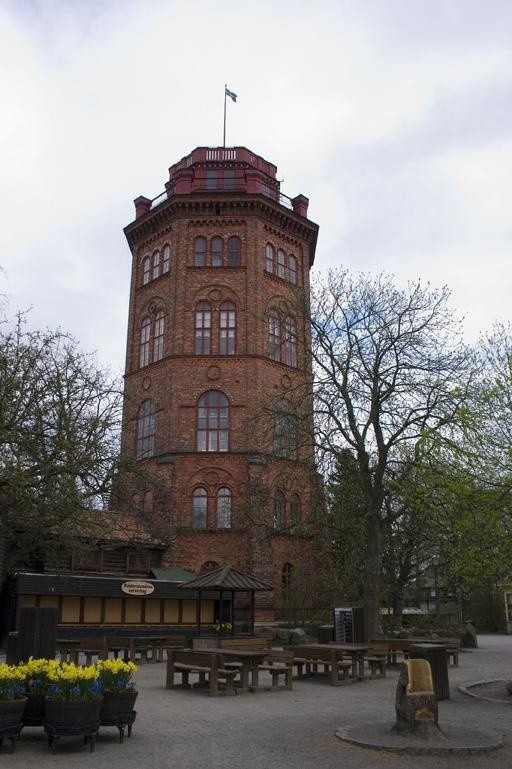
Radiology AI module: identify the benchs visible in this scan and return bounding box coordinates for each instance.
[56,635,462,697]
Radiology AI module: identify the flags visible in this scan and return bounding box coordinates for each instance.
[226,88,237,102]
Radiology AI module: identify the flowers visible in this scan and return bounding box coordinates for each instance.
[0,656,138,703]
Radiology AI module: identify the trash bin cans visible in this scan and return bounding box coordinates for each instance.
[410,644,449,701]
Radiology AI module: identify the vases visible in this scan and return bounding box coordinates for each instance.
[0,692,138,729]
